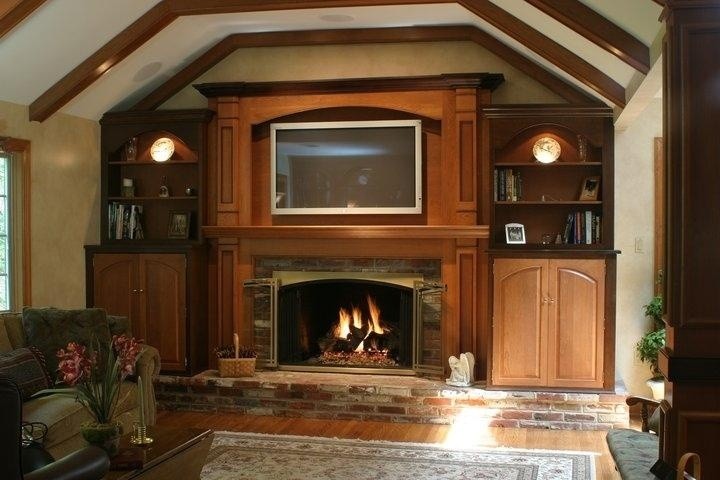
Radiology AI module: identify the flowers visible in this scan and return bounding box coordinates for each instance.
[29,334,143,425]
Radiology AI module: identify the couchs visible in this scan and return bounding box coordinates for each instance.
[1,377,109,479]
[1,308,162,462]
[606,395,662,480]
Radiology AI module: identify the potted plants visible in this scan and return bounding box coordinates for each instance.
[634,328,669,401]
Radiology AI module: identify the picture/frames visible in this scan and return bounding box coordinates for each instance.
[504,223,526,245]
[578,175,602,200]
[167,208,191,240]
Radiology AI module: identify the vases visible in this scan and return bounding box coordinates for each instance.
[80,420,123,458]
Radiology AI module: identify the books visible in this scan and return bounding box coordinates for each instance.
[494,168,523,202]
[106,201,144,240]
[562,211,601,245]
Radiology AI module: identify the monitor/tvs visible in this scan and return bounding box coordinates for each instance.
[269,120,423,216]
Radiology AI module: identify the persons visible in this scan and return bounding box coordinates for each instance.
[584,181,597,199]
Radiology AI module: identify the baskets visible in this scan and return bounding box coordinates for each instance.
[217,333,256,378]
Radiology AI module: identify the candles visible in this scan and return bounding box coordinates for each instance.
[136,375,144,426]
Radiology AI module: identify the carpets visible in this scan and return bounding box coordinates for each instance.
[197,429,597,480]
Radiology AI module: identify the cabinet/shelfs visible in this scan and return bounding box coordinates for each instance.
[81,109,214,375]
[480,102,623,393]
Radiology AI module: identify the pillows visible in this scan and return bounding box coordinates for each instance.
[22,304,113,389]
[1,345,50,405]
